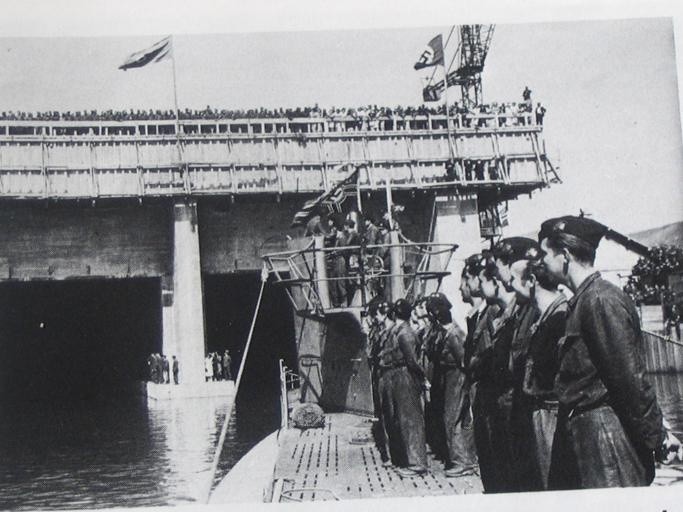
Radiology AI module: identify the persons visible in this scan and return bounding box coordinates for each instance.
[462,154,474,181]
[487,152,504,181]
[144,349,235,385]
[474,157,486,181]
[301,209,400,309]
[446,157,459,181]
[364,292,480,479]
[456,215,681,495]
[1,83,547,143]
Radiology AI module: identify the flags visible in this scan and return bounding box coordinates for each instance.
[116,33,174,73]
[412,33,446,70]
[290,168,358,227]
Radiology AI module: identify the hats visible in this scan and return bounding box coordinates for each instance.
[538,216,608,248]
[493,237,543,263]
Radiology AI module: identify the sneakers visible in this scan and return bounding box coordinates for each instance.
[445,463,474,477]
[382,459,427,478]
